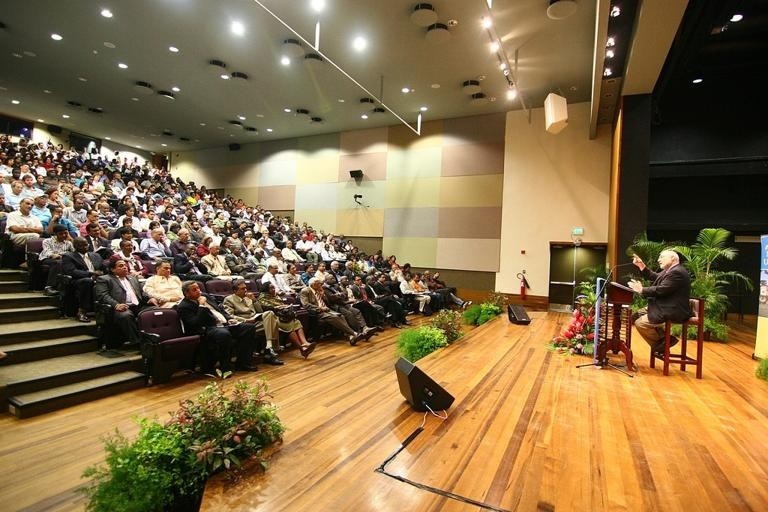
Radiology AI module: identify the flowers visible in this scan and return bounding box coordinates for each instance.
[546,295,609,358]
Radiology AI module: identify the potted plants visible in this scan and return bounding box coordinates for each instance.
[396,309,461,362]
[74,414,224,512]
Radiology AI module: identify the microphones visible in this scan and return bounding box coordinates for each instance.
[608,261,642,283]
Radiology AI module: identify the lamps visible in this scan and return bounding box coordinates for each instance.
[478,13,521,101]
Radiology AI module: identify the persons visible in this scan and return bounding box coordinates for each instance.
[627,250,696,359]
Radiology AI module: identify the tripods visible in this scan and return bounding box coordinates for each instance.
[576,265,633,377]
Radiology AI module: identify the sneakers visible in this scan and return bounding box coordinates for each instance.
[223,359,260,376]
[264,347,279,357]
[461,301,473,312]
[653,335,679,357]
[263,356,285,365]
[349,319,414,346]
[42,286,60,296]
[300,342,316,359]
[76,311,91,322]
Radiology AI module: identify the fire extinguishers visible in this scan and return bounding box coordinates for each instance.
[517,273,530,300]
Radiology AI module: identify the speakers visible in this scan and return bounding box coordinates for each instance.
[395,357,455,412]
[350,170,363,177]
[508,304,531,325]
[544,92,568,135]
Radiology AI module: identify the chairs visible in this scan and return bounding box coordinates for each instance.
[137,231,147,239]
[295,262,307,275]
[92,281,132,345]
[138,307,202,388]
[195,279,262,293]
[284,295,310,341]
[45,260,70,319]
[649,295,704,380]
[143,261,158,275]
[25,239,50,274]
